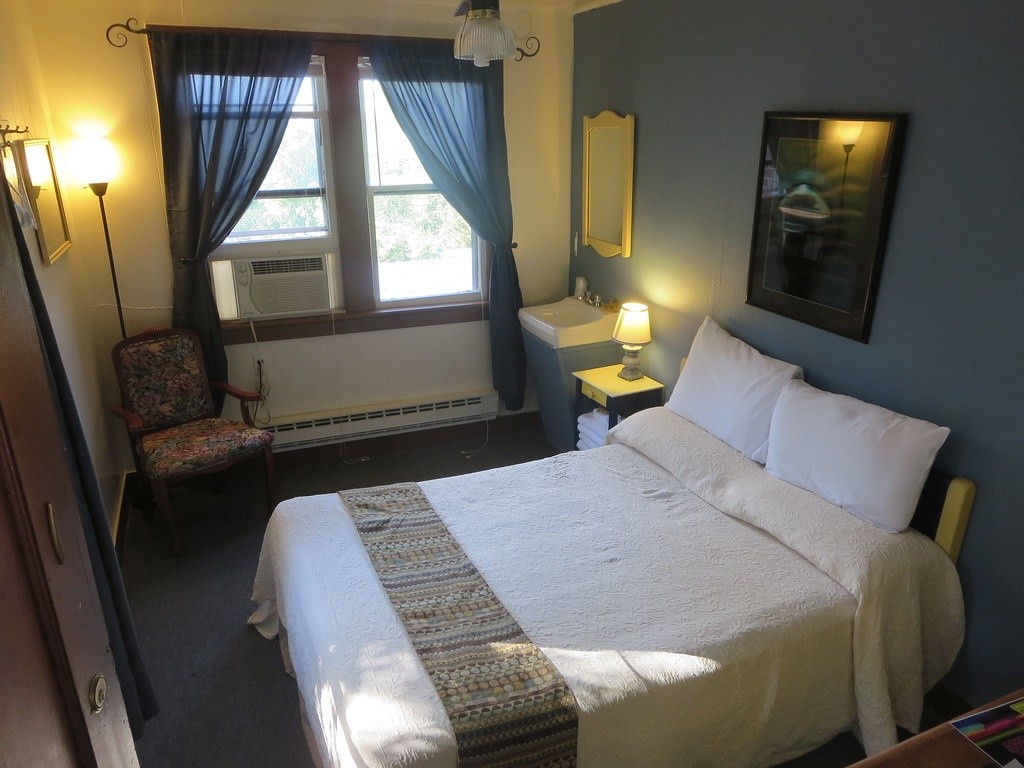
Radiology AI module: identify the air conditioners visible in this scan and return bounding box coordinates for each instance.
[231,254,330,319]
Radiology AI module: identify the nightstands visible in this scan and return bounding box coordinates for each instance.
[572,364,665,452]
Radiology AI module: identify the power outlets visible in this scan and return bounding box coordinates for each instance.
[252,355,268,376]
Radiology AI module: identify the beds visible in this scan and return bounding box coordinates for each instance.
[245,358,976,768]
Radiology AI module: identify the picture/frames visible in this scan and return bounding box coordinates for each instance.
[17,137,72,266]
[743,110,910,344]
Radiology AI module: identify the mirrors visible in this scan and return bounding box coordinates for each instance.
[581,111,634,258]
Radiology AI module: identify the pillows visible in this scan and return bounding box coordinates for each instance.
[666,317,805,466]
[765,379,951,534]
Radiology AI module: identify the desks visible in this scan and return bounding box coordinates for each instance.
[847,688,1024,768]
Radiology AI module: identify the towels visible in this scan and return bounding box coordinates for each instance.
[576,408,625,451]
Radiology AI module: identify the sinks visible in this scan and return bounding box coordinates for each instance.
[518,296,620,349]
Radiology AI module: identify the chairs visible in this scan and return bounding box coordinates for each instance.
[110,327,278,555]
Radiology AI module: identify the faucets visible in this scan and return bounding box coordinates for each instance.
[577,291,601,306]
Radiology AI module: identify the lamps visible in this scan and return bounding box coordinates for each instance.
[610,303,652,381]
[453,0,540,68]
[73,135,127,341]
[838,121,864,210]
